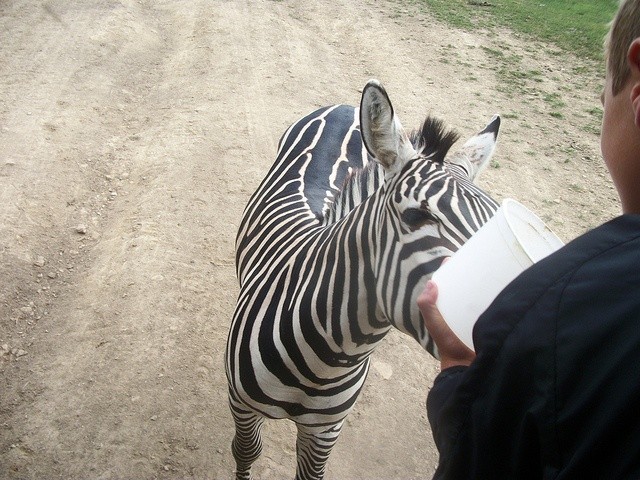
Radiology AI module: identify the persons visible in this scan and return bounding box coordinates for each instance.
[416,0,639,475]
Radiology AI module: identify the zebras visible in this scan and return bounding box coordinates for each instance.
[222,76,504,477]
